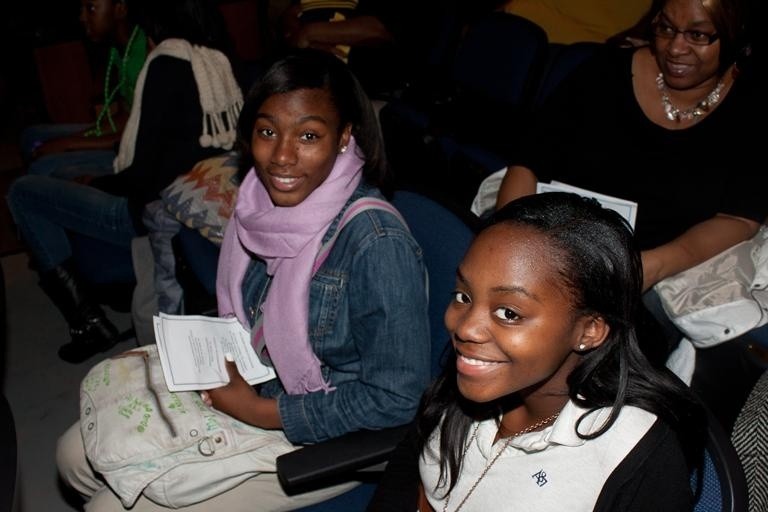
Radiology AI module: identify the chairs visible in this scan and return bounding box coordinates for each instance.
[276,190,491,507]
[666,220,767,509]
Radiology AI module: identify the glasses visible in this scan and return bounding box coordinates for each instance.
[653,24,720,47]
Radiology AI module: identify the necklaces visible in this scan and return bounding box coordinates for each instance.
[442,411,560,512]
[654,71,725,121]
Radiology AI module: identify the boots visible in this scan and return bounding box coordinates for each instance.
[39,272,119,364]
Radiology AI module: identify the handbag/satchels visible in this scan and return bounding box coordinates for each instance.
[78,344,303,509]
[652,224,768,348]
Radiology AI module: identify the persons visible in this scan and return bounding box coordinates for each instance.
[16,0,155,271]
[491,0,768,391]
[364,191,705,512]
[5,0,238,366]
[53,47,433,512]
